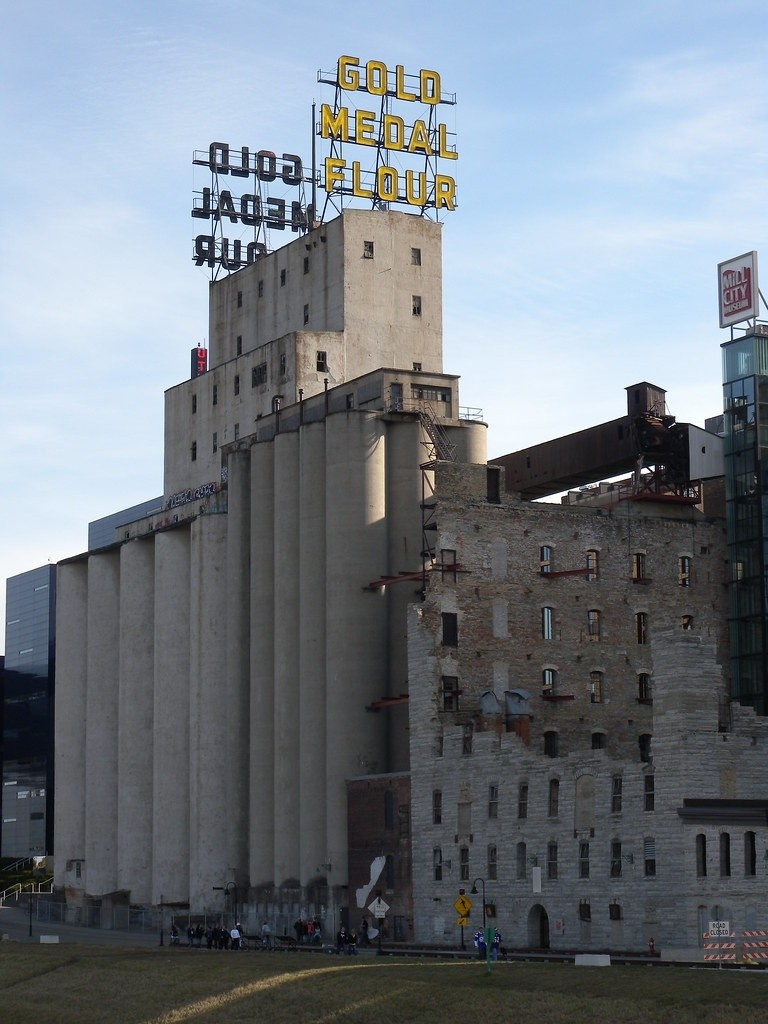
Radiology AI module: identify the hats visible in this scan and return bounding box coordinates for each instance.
[237,923,241,926]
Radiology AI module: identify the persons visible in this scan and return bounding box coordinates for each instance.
[336,926,360,956]
[473,926,504,961]
[294,916,322,945]
[360,915,369,945]
[170,922,244,950]
[261,921,272,943]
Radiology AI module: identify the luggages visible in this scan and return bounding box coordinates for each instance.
[500,942,507,957]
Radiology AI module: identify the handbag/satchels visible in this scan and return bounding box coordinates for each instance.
[171,935,173,938]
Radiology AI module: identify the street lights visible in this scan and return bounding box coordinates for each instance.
[225,881,238,925]
[469,878,486,927]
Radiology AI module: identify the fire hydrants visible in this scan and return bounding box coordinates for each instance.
[648,937,655,956]
